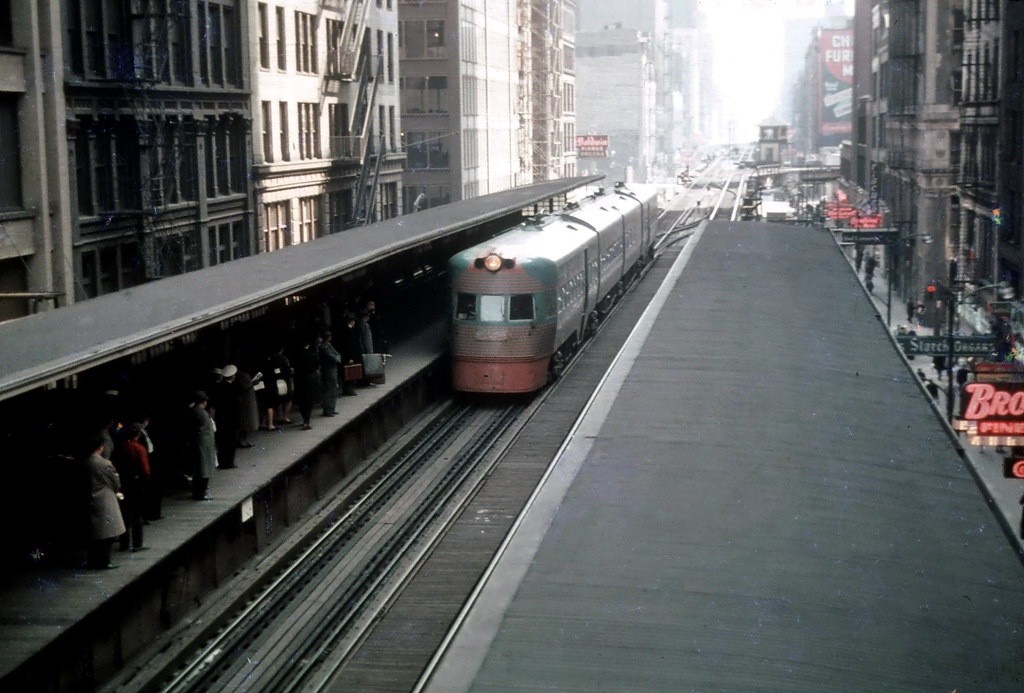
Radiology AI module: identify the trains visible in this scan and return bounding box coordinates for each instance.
[445,180,657,395]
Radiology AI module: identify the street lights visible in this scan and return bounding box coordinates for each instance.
[947,281,1017,426]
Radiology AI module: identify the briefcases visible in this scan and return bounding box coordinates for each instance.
[343,362,362,381]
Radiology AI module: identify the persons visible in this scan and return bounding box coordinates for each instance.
[82,302,388,570]
[864,256,877,281]
[894,297,969,402]
[807,200,826,223]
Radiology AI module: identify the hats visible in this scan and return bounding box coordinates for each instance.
[223,364,238,377]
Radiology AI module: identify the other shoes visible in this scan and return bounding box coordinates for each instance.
[239,440,256,448]
[324,411,339,417]
[266,426,281,432]
[194,492,215,501]
[218,463,238,470]
[302,424,312,430]
[279,416,294,424]
[91,563,119,570]
[132,545,150,552]
[119,545,130,551]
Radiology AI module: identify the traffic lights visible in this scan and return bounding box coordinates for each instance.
[924,284,938,299]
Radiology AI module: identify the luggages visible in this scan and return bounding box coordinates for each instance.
[361,353,385,383]
